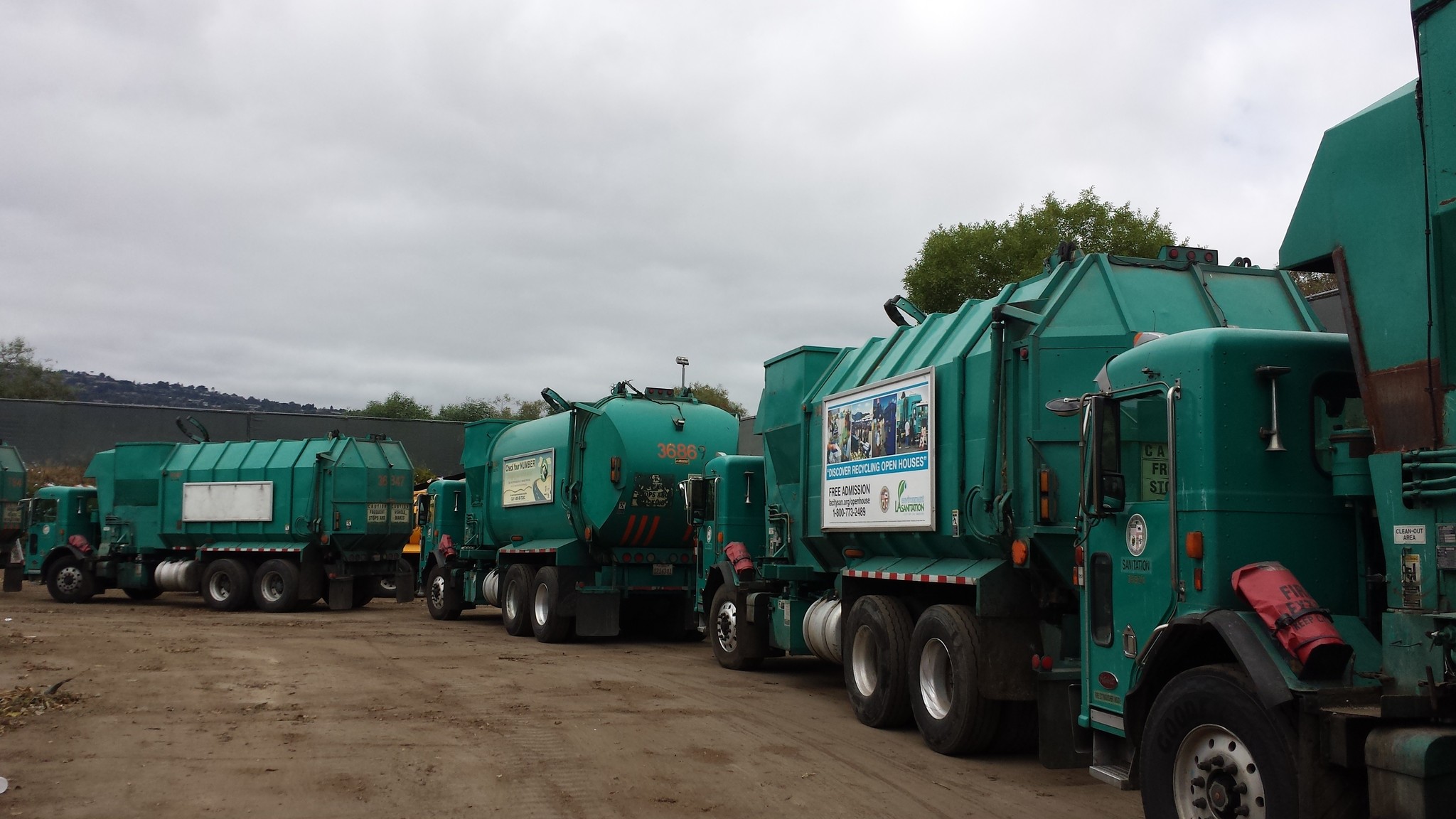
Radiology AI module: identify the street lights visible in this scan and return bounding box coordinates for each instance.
[675,354,691,394]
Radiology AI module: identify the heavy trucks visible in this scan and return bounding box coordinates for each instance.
[411,380,745,641]
[1058,0,1456,819]
[17,414,419,612]
[679,241,1328,760]
[0,442,29,593]
